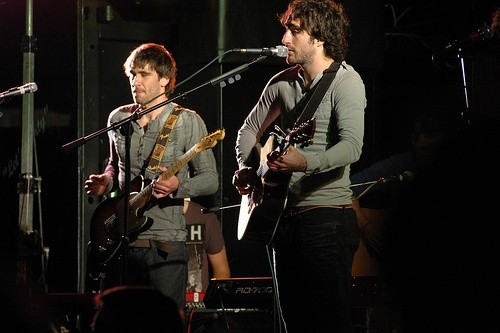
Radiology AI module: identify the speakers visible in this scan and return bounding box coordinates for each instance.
[0,286,188,333]
[204,280,276,309]
[189,308,273,333]
[349,274,413,333]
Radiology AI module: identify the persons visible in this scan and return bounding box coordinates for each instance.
[232,1,367,333]
[84,44,240,333]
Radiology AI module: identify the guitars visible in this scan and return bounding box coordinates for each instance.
[237,116,317,244]
[87,129,225,267]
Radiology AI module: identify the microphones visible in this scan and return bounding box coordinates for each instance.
[445,27,494,49]
[378,173,404,185]
[0,82,38,97]
[234,45,289,57]
